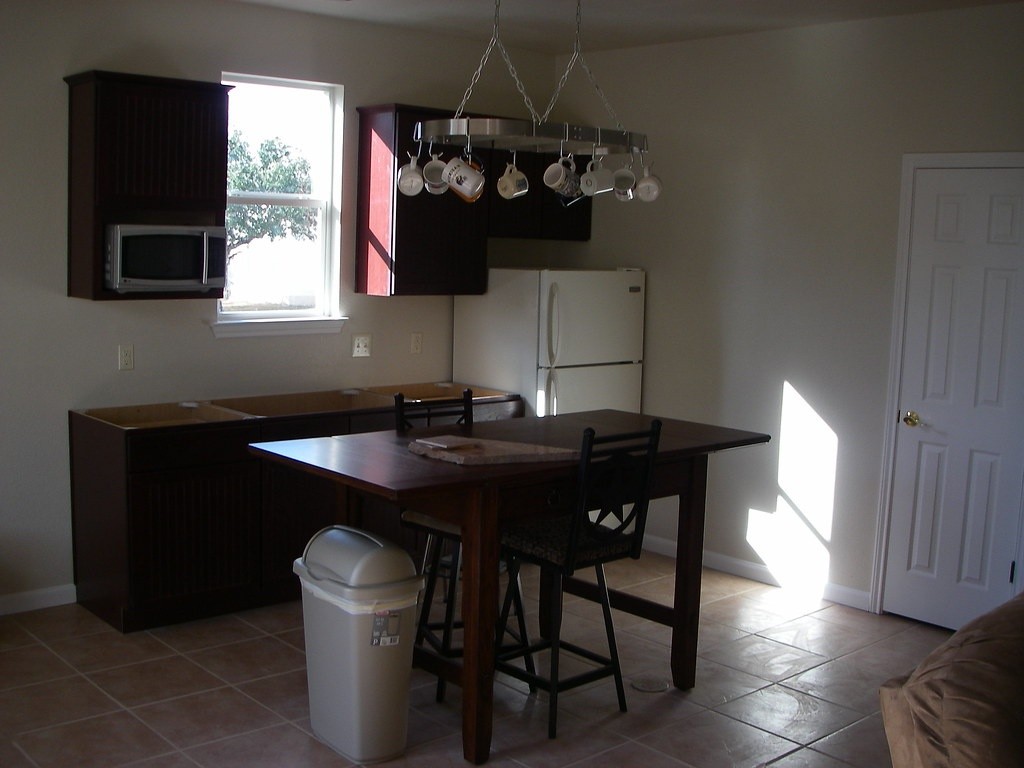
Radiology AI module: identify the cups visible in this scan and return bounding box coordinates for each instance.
[544,157,581,197]
[581,160,615,197]
[610,168,636,201]
[423,155,449,195]
[397,156,423,196]
[450,161,483,203]
[441,154,485,197]
[497,165,528,200]
[637,167,662,203]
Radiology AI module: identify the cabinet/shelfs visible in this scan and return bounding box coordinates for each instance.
[68,380,523,634]
[62,68,236,302]
[354,102,597,298]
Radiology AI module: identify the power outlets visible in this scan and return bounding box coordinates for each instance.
[410,332,423,354]
[351,332,372,357]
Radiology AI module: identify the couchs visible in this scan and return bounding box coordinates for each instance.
[879,591,1024,768]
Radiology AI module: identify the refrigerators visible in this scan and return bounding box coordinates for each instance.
[452,269,646,535]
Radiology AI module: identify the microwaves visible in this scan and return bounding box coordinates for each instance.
[105,225,226,292]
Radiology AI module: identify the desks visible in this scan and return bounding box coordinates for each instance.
[246,407,771,766]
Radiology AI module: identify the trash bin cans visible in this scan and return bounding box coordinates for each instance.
[292,524,426,766]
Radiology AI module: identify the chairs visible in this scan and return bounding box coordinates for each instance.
[392,389,539,707]
[494,417,665,739]
